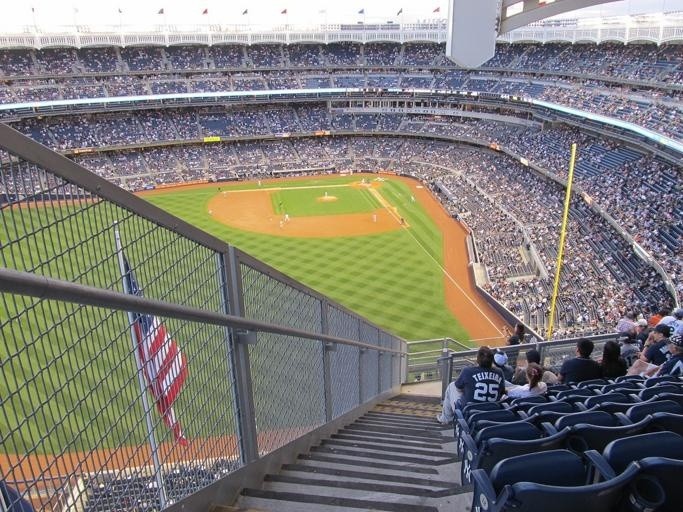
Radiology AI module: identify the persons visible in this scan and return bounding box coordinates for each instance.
[398,218,407,225]
[324,191,327,198]
[409,193,416,203]
[207,208,212,216]
[413,374,422,382]
[278,218,284,232]
[84,458,249,512]
[284,213,289,223]
[267,216,272,224]
[278,199,281,206]
[372,213,376,223]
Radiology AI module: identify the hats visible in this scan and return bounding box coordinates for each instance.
[665,334,683,349]
[493,351,508,367]
[634,318,648,327]
[648,323,670,336]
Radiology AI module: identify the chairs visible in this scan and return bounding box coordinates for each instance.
[449,366,683,510]
[83,461,244,512]
[444,42,683,344]
[1,40,441,201]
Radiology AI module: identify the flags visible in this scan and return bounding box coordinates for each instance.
[112,222,189,447]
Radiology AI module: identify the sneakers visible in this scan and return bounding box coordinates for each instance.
[436,414,449,425]
